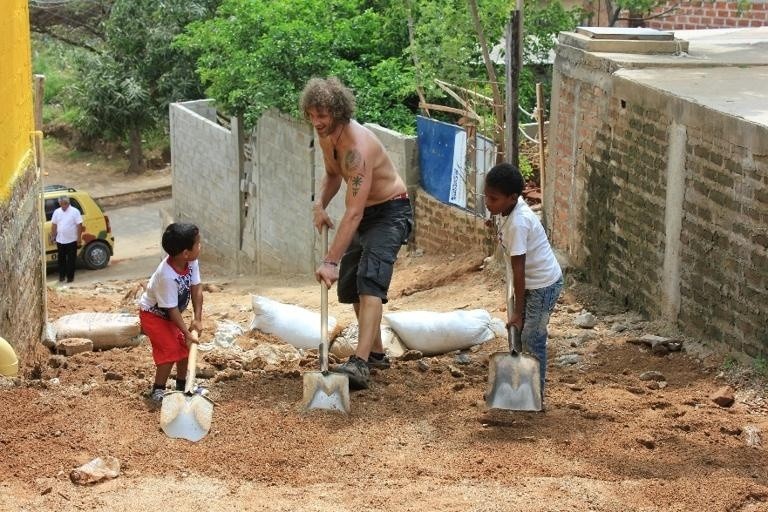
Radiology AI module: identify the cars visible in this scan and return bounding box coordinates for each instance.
[39,186,114,269]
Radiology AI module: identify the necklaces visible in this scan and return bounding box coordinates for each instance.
[495,211,510,235]
[327,123,346,162]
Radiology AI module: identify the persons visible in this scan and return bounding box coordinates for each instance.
[300,75,415,390]
[140,221,203,399]
[484,162,564,408]
[51,195,82,282]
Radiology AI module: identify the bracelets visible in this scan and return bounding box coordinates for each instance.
[319,259,338,267]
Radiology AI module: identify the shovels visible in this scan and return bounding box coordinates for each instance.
[160,310,216,443]
[484,251,542,412]
[302,223,352,414]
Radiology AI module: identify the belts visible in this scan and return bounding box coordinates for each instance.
[364,198,411,216]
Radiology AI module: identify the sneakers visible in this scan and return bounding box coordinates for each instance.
[176,385,209,397]
[335,356,371,390]
[349,353,391,370]
[150,390,165,401]
